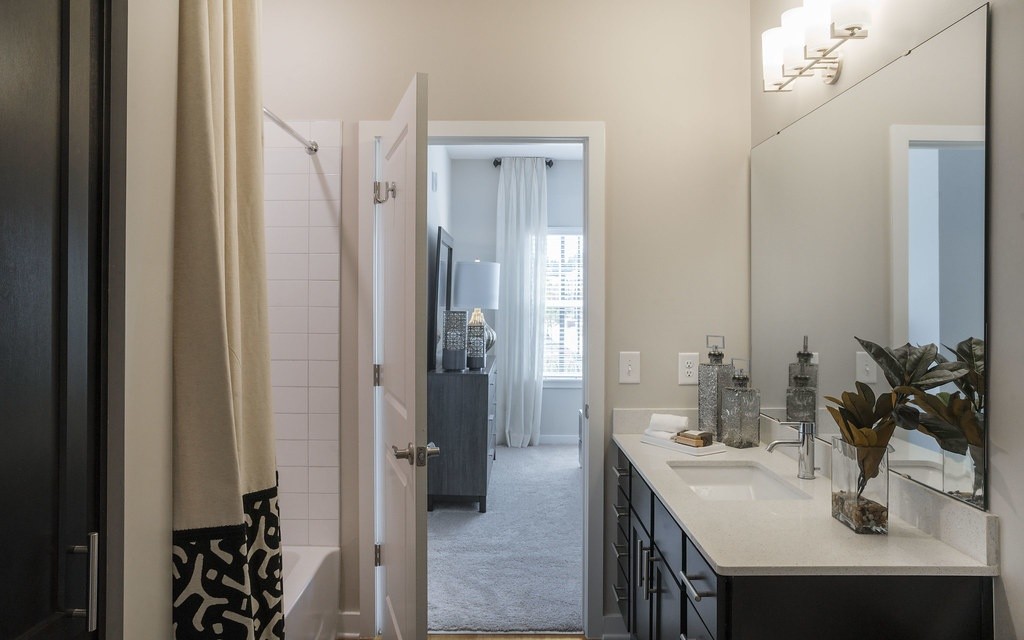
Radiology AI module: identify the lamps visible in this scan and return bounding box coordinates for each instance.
[452,258,500,352]
[762,0,872,92]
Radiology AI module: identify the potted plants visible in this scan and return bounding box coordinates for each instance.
[824,336,937,534]
[916,338,985,509]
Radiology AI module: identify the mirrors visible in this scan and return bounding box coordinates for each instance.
[432,227,454,369]
[750,3,992,512]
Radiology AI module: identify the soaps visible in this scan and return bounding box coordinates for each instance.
[674,437,704,448]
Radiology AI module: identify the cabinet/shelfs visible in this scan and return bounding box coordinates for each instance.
[427,355,496,512]
[611,448,994,640]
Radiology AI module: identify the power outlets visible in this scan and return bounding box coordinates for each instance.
[678,353,700,385]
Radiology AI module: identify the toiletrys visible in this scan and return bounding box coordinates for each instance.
[785,360,818,430]
[720,367,761,449]
[694,334,735,443]
[788,333,817,392]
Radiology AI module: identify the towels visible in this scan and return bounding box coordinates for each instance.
[648,412,690,433]
[643,428,678,438]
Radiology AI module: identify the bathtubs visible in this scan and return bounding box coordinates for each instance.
[282,544,344,640]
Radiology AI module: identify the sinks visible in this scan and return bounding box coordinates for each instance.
[883,456,986,503]
[666,458,814,505]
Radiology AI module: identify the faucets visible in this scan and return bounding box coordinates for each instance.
[764,415,819,480]
